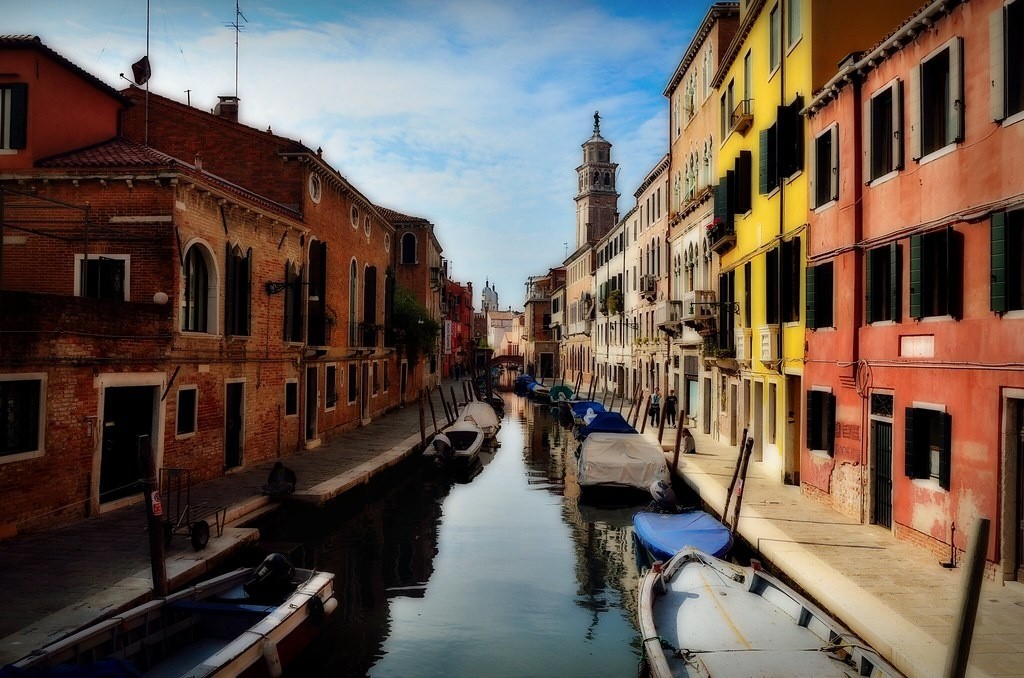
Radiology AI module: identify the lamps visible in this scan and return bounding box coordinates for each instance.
[689,301,740,315]
[266,282,318,301]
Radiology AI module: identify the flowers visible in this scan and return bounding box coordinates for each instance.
[706,218,720,230]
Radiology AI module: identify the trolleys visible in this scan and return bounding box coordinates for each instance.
[158,467,211,551]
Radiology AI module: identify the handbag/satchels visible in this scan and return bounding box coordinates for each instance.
[649,407,655,416]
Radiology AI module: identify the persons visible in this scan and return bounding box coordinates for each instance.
[651,386,662,428]
[681,428,696,454]
[665,390,678,429]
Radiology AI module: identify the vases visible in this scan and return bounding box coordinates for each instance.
[707,225,721,237]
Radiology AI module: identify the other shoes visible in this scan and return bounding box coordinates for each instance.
[673,425,676,428]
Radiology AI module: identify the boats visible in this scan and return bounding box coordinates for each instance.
[21,540,341,678]
[577,433,670,492]
[453,362,505,441]
[549,386,586,407]
[570,402,639,441]
[633,480,734,568]
[421,415,486,480]
[638,545,905,678]
[514,374,552,397]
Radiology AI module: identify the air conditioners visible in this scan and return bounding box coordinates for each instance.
[639,274,656,294]
